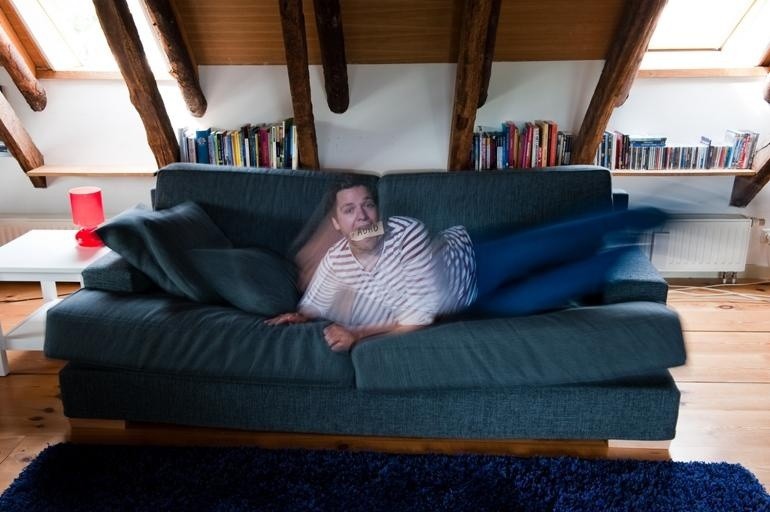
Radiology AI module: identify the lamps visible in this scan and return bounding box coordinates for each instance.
[68,186,107,247]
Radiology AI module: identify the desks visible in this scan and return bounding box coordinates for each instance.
[0,226,111,377]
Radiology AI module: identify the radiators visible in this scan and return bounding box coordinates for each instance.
[639,212,751,285]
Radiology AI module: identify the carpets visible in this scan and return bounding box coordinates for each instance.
[0,442,768,511]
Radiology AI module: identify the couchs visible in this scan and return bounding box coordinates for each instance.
[44,160,683,446]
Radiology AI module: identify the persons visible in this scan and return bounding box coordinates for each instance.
[262,172,670,352]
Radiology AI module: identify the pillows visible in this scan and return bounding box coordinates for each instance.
[90,205,295,319]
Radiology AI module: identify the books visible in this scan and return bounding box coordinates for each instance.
[471,118,760,171]
[179,117,298,171]
